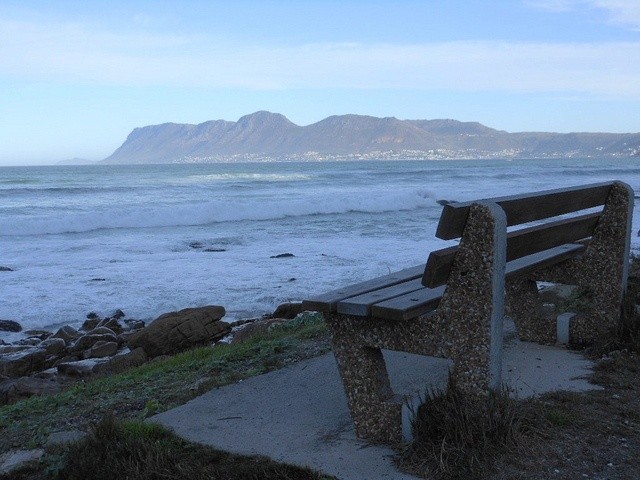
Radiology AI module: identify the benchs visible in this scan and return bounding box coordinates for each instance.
[301,179,635,442]
